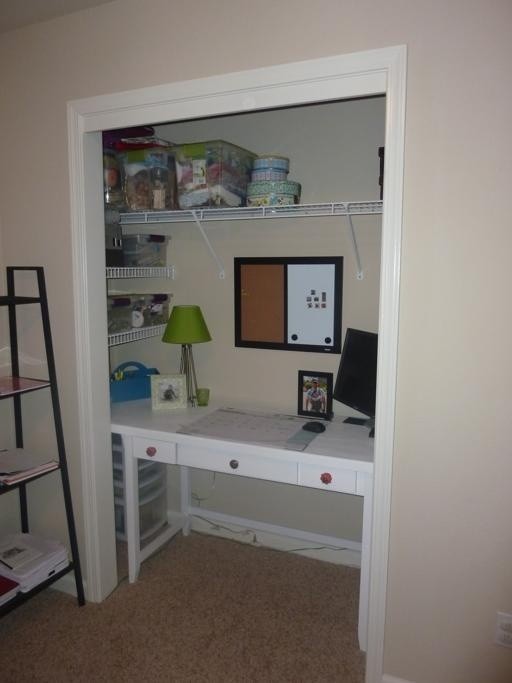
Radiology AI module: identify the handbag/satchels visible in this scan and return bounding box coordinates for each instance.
[110,361,160,403]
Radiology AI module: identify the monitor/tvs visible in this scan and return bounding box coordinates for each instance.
[333,327,378,437]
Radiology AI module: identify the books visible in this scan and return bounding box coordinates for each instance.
[0,531,71,607]
[0,446,62,486]
[1,376,51,396]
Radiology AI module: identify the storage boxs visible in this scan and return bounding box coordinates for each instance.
[123,234,173,265]
[114,142,178,210]
[167,138,260,207]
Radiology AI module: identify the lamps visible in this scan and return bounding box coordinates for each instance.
[161,302,214,406]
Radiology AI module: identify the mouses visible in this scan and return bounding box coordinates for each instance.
[303,421,326,432]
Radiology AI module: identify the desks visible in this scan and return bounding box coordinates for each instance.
[110,394,374,655]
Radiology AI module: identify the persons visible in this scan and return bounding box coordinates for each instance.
[304,378,327,411]
[165,383,177,400]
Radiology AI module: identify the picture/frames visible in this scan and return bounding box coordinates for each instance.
[296,368,334,421]
[150,374,189,411]
[231,253,344,354]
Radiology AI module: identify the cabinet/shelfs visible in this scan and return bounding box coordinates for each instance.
[113,436,168,544]
[105,262,175,346]
[0,263,86,623]
